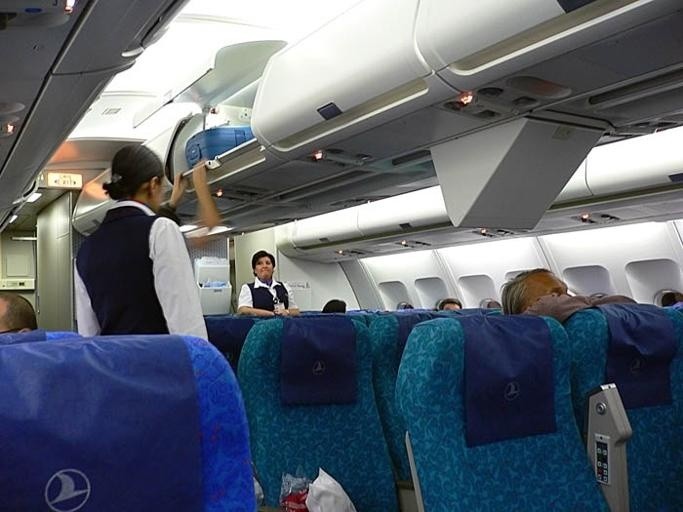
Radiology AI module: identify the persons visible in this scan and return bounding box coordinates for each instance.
[439,299,462,310]
[322,300,346,313]
[157,165,219,245]
[501,268,636,324]
[237,251,299,318]
[0,294,37,334]
[74,144,209,342]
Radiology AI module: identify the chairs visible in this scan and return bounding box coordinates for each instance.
[568,303,682,508]
[238,316,400,512]
[0,333,257,510]
[442,306,503,317]
[394,315,612,512]
[290,310,379,324]
[372,312,436,480]
[0,330,82,347]
[204,317,256,362]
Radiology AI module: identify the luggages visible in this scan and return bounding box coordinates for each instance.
[185,128,251,170]
